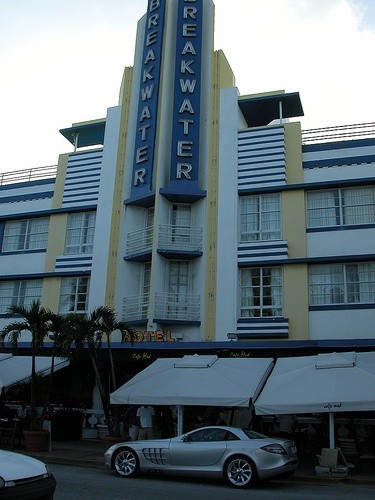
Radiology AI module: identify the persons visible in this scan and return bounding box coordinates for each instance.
[124,404,156,441]
[17,403,28,418]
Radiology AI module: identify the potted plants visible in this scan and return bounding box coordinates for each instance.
[0,301,65,452]
[49,306,138,449]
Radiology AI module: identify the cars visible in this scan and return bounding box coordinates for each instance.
[0,451,57,500]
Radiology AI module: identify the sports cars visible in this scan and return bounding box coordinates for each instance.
[105,426,299,488]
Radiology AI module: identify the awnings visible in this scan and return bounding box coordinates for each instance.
[0,351,72,396]
[254,350,375,414]
[108,353,274,408]
